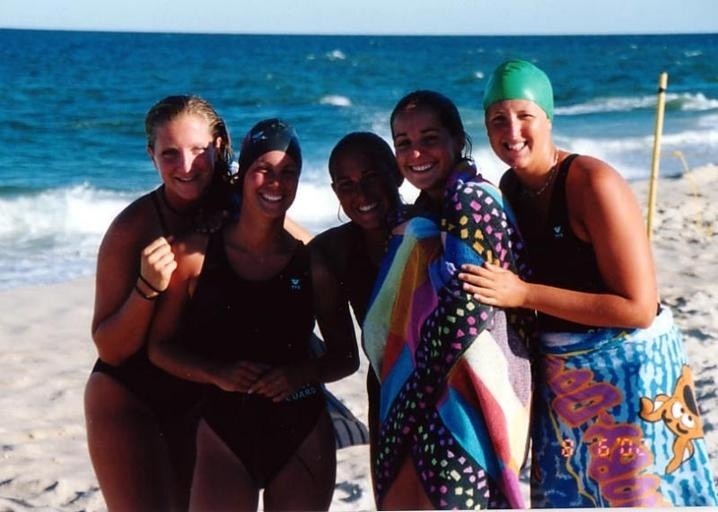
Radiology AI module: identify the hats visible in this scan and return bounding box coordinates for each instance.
[238,119,301,180]
[482,60,554,121]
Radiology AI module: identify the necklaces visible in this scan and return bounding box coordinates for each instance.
[157,183,184,218]
[523,148,559,199]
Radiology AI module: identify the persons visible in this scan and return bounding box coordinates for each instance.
[82,94,314,511]
[357,90,537,510]
[458,59,718,509]
[191,132,414,504]
[144,117,360,511]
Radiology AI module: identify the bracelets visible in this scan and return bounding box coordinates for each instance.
[139,274,163,294]
[134,283,158,300]
